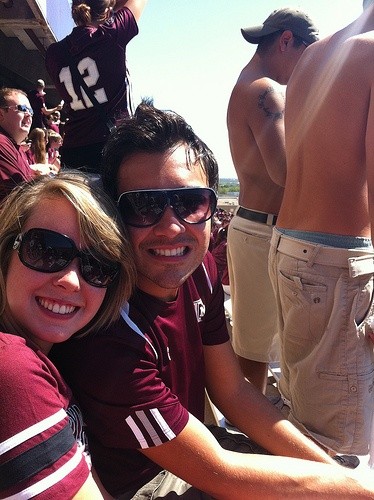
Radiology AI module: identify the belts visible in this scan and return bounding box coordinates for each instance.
[236,208,278,225]
[270,226,373,278]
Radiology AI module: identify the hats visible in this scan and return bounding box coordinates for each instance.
[241,8,318,44]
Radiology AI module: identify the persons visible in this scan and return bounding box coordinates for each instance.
[268,0,374,470]
[0,167,139,500]
[224,6,320,428]
[0,79,65,200]
[65,98,374,500]
[44,0,147,173]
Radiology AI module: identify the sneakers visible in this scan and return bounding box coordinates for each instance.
[334,455,361,470]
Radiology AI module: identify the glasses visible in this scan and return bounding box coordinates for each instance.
[115,188,219,226]
[2,104,33,115]
[13,228,122,288]
